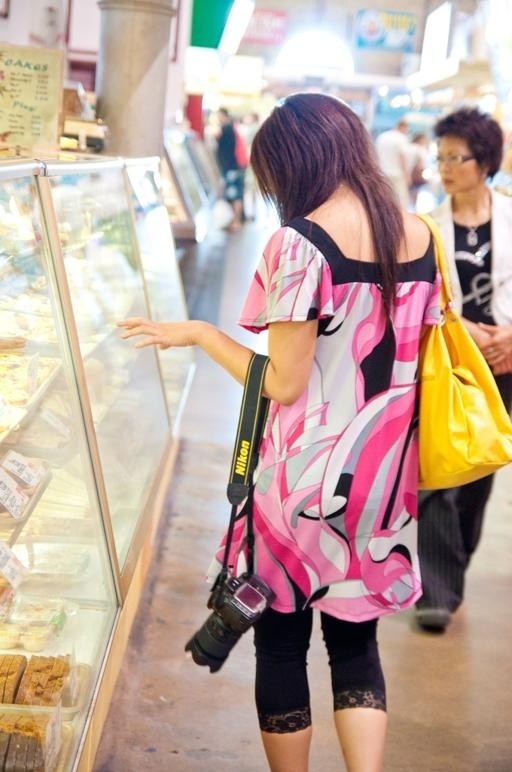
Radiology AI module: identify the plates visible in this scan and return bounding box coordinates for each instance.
[0,148,203,772]
[153,115,233,247]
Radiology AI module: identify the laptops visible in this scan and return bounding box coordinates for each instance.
[0,653,72,772]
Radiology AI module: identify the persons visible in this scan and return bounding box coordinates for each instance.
[416,105,512,630]
[413,132,430,196]
[206,108,262,237]
[375,120,415,208]
[119,94,438,771]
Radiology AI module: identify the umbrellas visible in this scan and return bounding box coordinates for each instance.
[416,608,456,626]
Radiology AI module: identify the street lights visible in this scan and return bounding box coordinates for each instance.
[416,211,512,492]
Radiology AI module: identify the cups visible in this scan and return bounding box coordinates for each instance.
[435,153,478,164]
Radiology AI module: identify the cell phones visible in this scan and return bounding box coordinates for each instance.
[185,570,276,673]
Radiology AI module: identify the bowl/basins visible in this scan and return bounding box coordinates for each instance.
[465,224,479,247]
